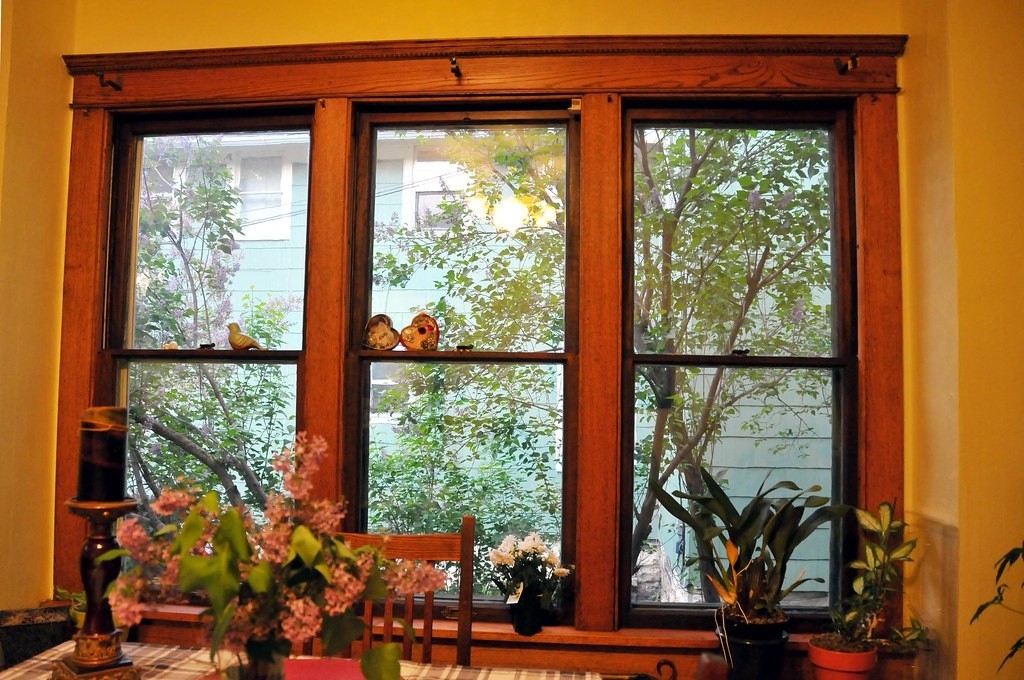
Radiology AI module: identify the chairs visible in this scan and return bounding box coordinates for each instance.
[302,514,475,665]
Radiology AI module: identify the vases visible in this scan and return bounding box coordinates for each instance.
[508,596,543,636]
[242,637,284,680]
[0,608,71,671]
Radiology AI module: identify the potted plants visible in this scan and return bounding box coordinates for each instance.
[649,466,936,680]
[55,584,130,642]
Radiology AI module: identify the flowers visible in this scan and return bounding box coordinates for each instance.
[487,533,575,605]
[94,427,450,680]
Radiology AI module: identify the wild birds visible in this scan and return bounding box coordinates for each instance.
[227,322,268,350]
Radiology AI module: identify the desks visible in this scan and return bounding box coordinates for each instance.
[0,636,649,680]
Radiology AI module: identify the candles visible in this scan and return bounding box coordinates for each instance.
[76,406,129,503]
